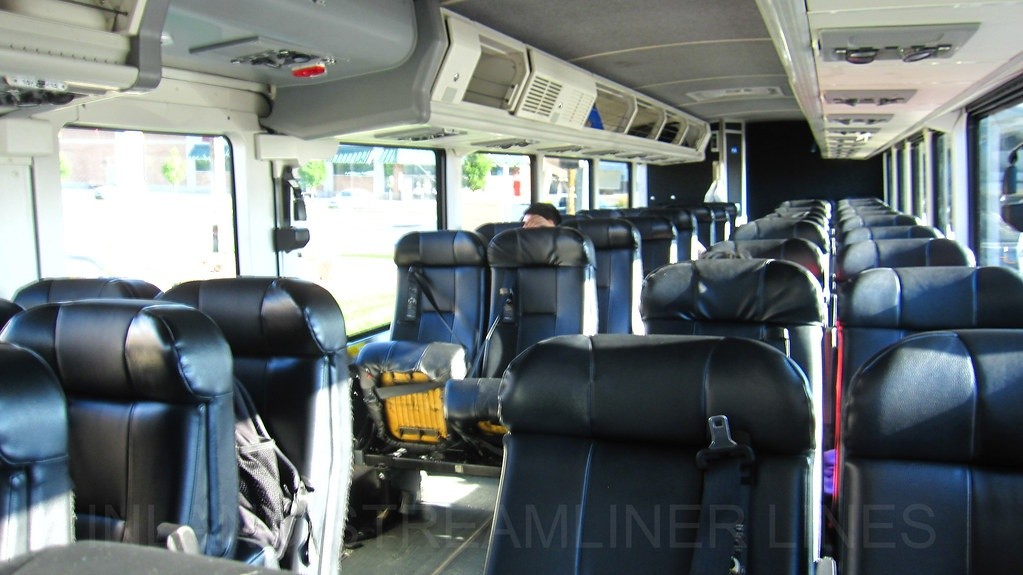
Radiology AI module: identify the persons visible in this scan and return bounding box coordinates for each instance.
[522,203,562,229]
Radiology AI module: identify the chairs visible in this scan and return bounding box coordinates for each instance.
[483,195,1023,575]
[0,201,730,574]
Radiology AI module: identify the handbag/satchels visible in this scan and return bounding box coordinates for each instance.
[229,378,309,555]
